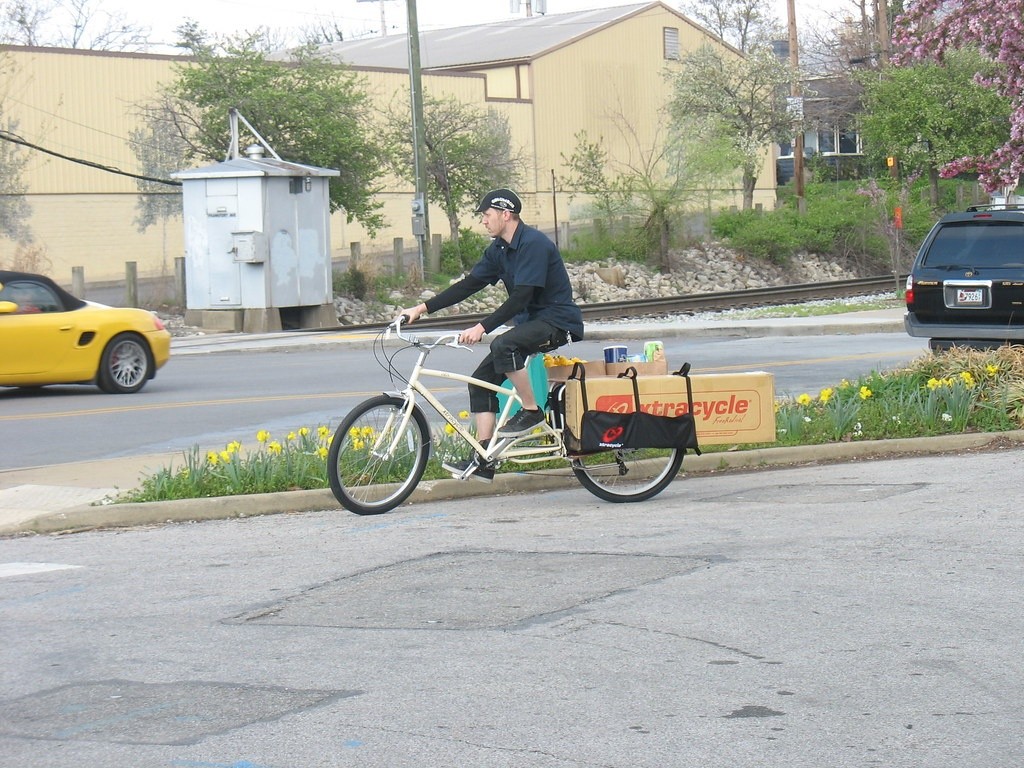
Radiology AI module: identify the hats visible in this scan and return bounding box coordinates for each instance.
[474,188,521,214]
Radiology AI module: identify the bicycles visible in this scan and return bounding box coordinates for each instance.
[327,314,687,516]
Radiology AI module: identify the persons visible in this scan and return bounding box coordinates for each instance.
[19,295,39,314]
[400,189,584,485]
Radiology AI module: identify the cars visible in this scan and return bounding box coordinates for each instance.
[0,270,171,395]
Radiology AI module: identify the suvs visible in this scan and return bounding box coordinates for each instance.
[904,204,1023,355]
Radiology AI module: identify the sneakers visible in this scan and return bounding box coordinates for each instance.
[442,459,495,483]
[497,405,546,437]
